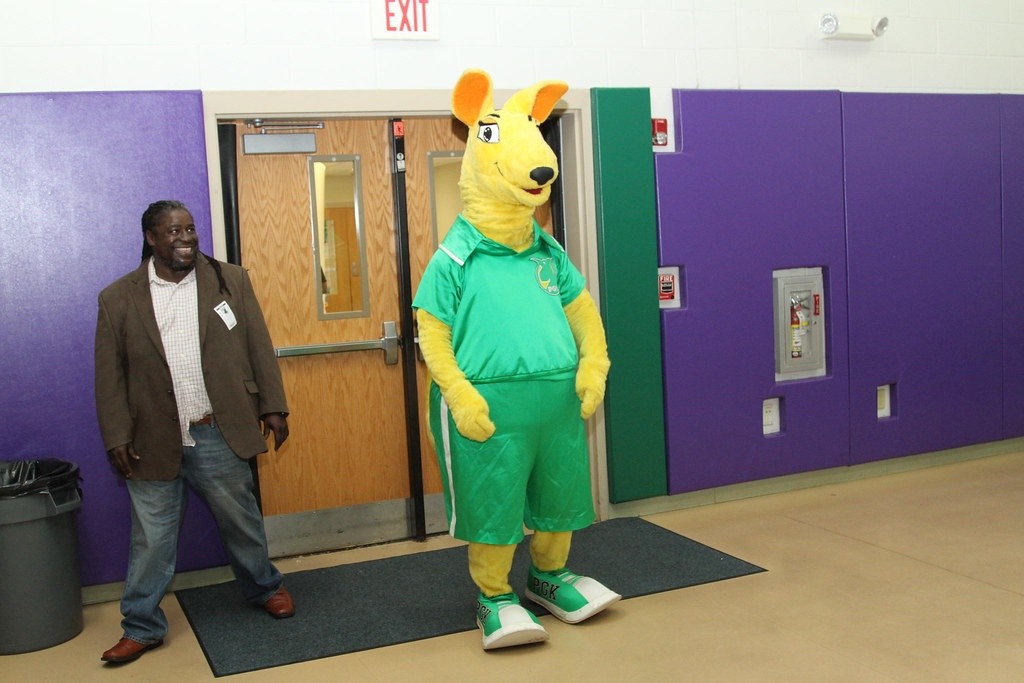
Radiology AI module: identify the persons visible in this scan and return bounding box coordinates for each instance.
[94,201,295,662]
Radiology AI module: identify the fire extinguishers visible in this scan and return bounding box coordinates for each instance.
[791,296,813,358]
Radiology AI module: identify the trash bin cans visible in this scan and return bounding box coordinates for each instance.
[0,457,84,657]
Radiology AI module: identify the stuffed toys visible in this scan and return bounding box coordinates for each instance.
[412,69,622,650]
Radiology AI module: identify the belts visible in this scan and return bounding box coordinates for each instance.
[190,415,211,426]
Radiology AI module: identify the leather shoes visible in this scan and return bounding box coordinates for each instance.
[264,585,294,619]
[101,637,163,662]
